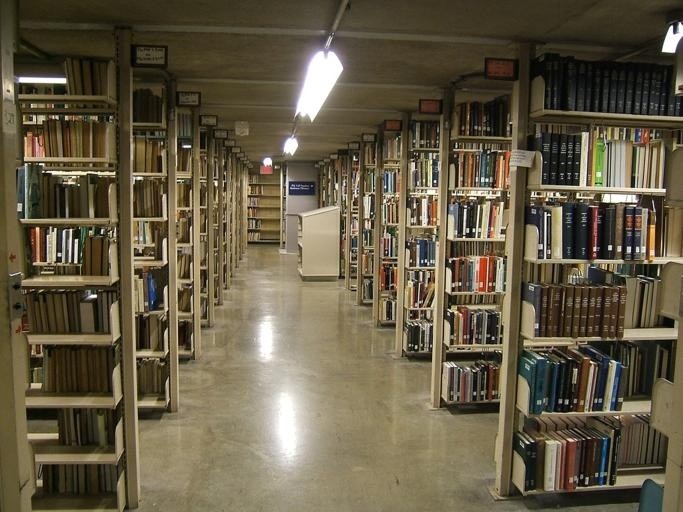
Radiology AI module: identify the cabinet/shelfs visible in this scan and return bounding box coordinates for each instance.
[296,205,342,283]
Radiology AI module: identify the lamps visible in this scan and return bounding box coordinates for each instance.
[661,16,683,52]
[291,48,345,124]
[282,137,299,157]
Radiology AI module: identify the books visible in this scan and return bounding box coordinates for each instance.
[16,53,123,496]
[515,55,682,495]
[130,85,169,393]
[177,112,194,346]
[322,143,374,300]
[199,132,264,317]
[380,93,514,402]
